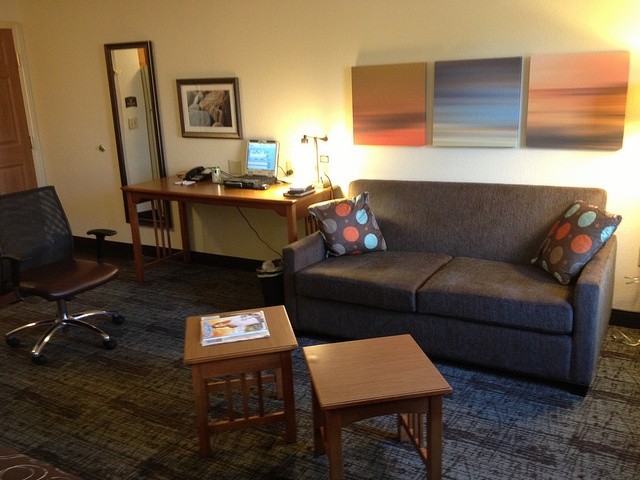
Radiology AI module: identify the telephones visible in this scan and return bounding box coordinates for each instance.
[184,166,213,182]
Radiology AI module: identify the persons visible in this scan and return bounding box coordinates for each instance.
[211,315,260,329]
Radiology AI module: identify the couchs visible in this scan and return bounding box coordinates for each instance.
[282,178,618,397]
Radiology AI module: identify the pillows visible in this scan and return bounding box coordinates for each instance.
[306,191,385,256]
[532,199,623,286]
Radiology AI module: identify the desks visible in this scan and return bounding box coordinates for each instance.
[120,171,338,281]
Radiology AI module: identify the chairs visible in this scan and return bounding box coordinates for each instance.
[0,184,125,365]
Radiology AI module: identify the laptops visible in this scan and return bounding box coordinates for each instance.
[223,139,281,190]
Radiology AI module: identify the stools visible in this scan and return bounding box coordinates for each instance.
[183,303,300,456]
[301,333,453,479]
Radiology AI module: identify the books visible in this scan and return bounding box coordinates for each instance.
[283,183,316,198]
[198,310,271,346]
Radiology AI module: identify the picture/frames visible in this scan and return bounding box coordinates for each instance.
[176,77,243,139]
